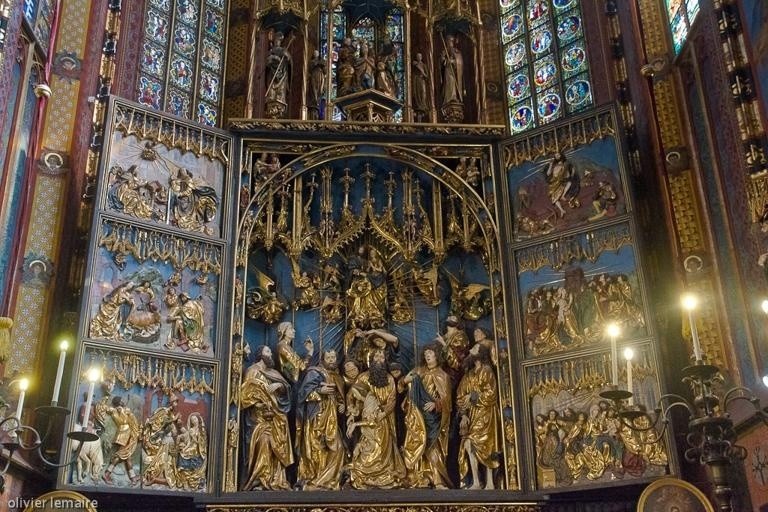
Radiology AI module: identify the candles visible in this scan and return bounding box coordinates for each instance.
[682,294,702,361]
[83,370,98,427]
[15,374,28,428]
[611,327,619,387]
[626,349,635,406]
[51,340,69,402]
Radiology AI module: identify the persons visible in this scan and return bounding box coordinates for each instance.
[265,35,464,112]
[71,151,666,490]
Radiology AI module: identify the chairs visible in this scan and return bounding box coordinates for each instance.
[22,491,97,512]
[637,478,714,512]
